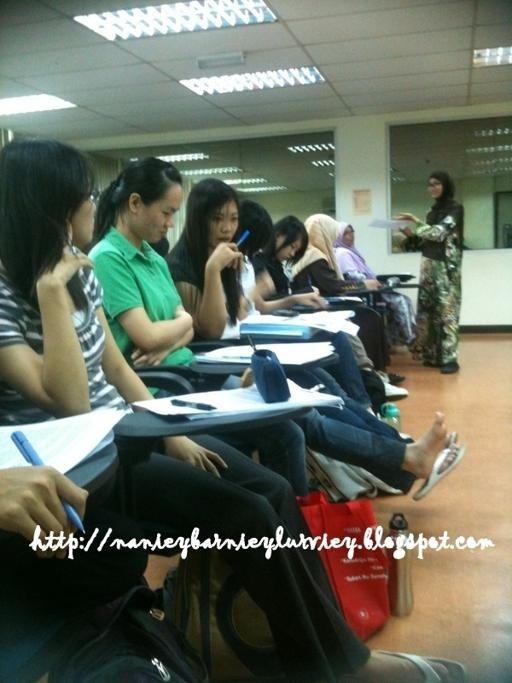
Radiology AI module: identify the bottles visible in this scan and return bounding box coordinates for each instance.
[380,401,401,434]
[386,512,414,616]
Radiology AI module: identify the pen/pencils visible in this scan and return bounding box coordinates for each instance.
[11,431,86,535]
[223,356,252,359]
[171,399,217,411]
[237,230,250,246]
[307,274,312,286]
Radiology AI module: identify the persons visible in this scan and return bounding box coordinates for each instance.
[0,136,470,682]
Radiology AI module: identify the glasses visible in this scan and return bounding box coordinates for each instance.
[429,181,440,187]
[80,189,99,202]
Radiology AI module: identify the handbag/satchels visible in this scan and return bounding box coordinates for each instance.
[298,491,390,641]
[49,585,207,683]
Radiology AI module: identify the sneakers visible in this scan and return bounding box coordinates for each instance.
[382,382,408,401]
[388,373,405,383]
[441,363,458,373]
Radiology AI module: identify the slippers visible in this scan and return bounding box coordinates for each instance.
[373,648,467,683]
[413,446,463,500]
